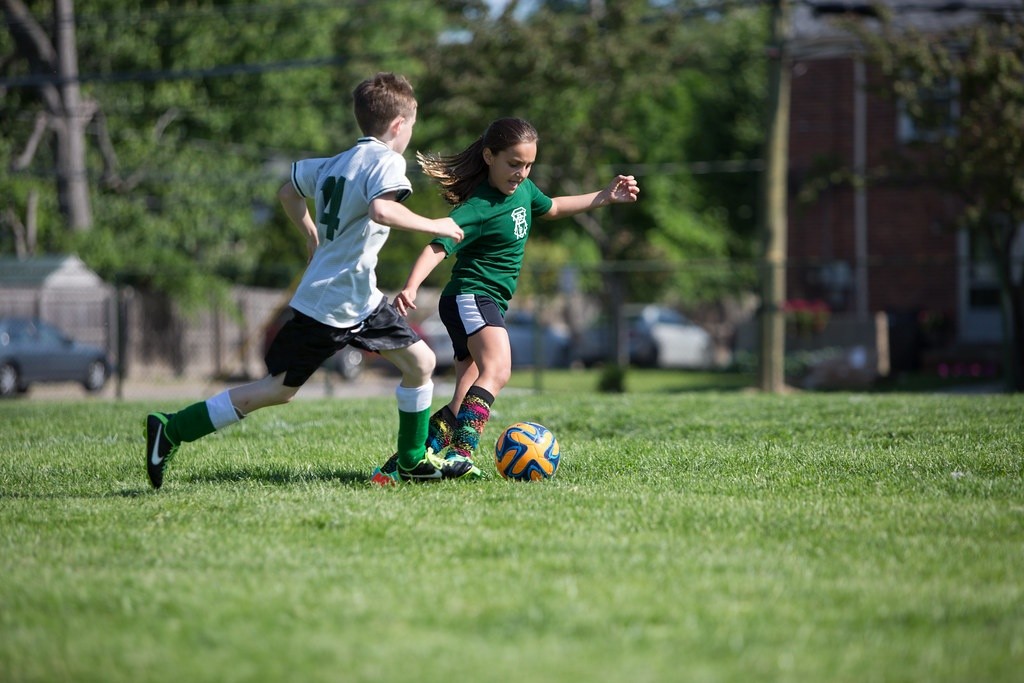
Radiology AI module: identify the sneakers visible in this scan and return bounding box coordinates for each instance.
[394,448,475,482]
[446,452,481,480]
[143,412,181,490]
[370,468,407,486]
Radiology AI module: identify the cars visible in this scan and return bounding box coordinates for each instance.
[574,303,735,372]
[0,315,112,398]
[261,293,571,380]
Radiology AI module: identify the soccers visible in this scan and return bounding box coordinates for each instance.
[494,421,562,482]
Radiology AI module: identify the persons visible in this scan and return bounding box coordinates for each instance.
[143,73,473,489]
[370,118,640,487]
